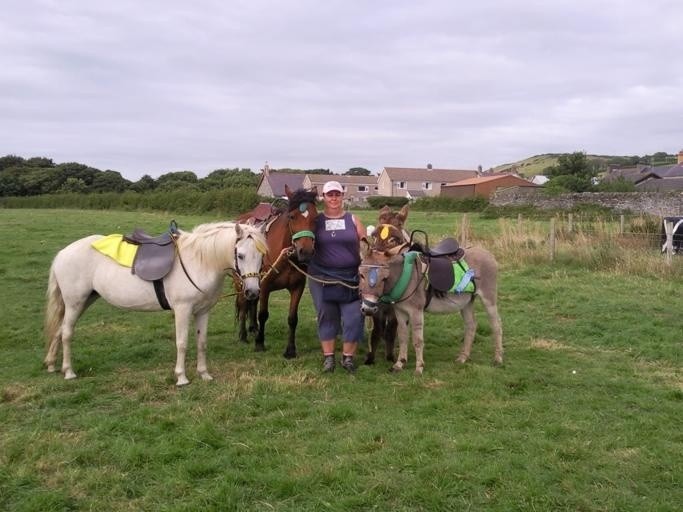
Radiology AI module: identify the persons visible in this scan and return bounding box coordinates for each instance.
[306,180,367,372]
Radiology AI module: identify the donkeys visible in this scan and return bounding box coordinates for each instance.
[365,204,410,365]
[358,237,503,377]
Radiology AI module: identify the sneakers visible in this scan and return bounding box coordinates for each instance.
[339,356,354,374]
[320,356,336,373]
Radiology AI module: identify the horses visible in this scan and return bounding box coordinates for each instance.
[234,184,318,359]
[43,222,270,390]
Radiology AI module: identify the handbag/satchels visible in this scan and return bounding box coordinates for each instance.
[322,273,359,303]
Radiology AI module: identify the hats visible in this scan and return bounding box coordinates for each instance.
[323,181,343,193]
